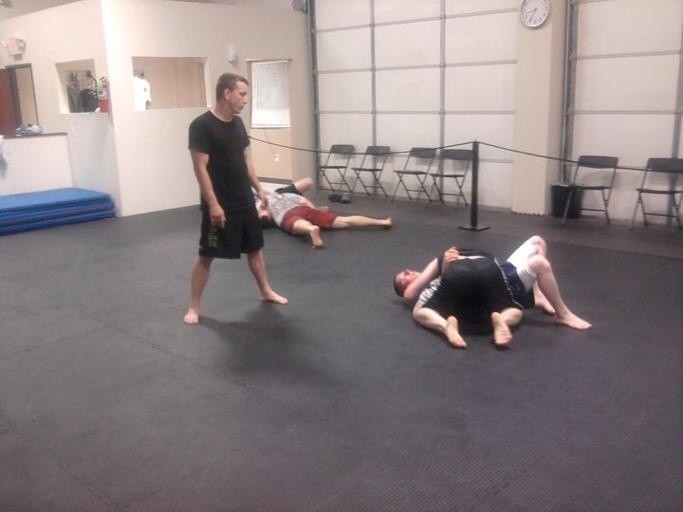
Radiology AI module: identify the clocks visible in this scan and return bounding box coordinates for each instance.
[519,0,552,28]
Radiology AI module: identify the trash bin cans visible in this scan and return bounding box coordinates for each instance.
[552,182,584,219]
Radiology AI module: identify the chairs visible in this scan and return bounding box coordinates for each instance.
[318,145,353,193]
[425,149,473,210]
[561,155,617,229]
[629,158,683,234]
[390,148,435,206]
[351,146,389,198]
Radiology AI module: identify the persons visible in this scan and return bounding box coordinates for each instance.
[393,235,593,348]
[133,68,152,111]
[183,73,288,324]
[253,177,393,249]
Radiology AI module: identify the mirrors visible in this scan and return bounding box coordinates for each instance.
[246,58,292,129]
